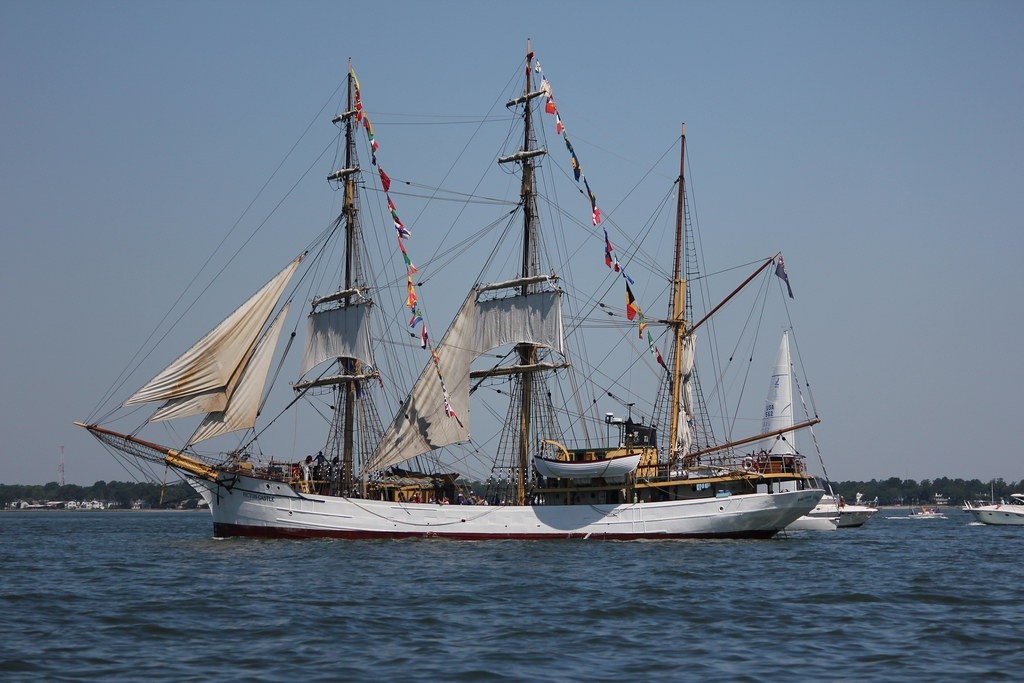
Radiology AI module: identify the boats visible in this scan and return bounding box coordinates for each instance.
[964,498,1024,525]
[884,509,948,520]
[839,501,878,527]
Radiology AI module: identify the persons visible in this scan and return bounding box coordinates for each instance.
[312,451,329,466]
[398,487,489,505]
[297,454,313,481]
[350,485,360,499]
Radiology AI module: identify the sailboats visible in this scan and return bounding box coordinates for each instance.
[73,37,825,538]
[756,329,845,530]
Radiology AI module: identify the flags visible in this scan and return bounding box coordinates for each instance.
[353,69,463,430]
[775,256,794,299]
[539,75,675,396]
